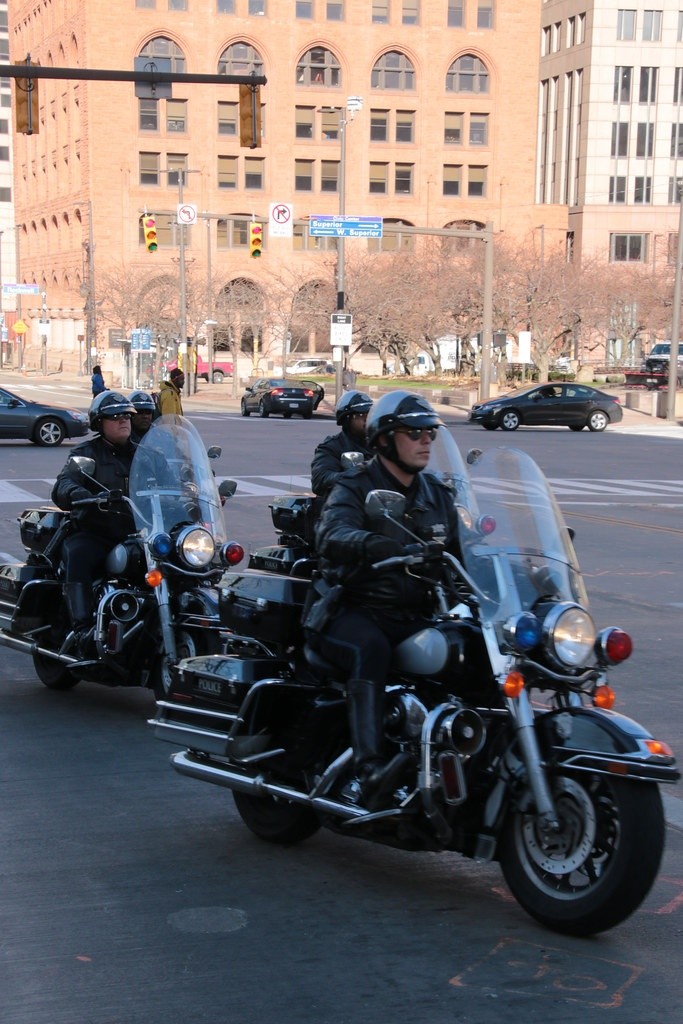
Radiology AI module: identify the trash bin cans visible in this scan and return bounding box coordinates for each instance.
[100,371,113,386]
[657,390,668,419]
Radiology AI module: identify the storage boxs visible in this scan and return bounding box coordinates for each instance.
[0,563,46,597]
[249,544,298,573]
[268,494,315,528]
[171,654,268,730]
[216,577,311,643]
[16,507,63,548]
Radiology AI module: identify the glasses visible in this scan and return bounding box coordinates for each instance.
[102,413,132,421]
[348,412,368,420]
[137,409,153,414]
[383,427,437,442]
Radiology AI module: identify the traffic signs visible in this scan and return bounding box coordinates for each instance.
[309,215,383,239]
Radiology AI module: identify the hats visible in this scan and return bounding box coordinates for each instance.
[171,368,184,380]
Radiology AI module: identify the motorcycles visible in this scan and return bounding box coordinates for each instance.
[0,414,244,700]
[147,446,682,936]
[247,425,496,574]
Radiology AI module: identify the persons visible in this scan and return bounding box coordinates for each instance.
[91,366,106,398]
[310,391,372,496]
[302,389,536,779]
[128,391,194,478]
[51,391,200,643]
[157,369,185,436]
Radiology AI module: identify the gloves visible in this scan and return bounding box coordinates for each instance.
[70,487,95,510]
[363,534,406,568]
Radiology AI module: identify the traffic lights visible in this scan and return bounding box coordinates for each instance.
[142,216,158,253]
[249,222,262,259]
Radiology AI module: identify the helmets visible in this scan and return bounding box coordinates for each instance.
[127,391,156,411]
[365,390,448,448]
[88,390,138,431]
[335,389,374,425]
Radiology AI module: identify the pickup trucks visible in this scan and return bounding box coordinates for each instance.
[146,355,234,384]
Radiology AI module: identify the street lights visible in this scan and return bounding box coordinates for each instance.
[160,168,200,397]
[317,109,344,406]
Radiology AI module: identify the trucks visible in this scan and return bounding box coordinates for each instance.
[385,329,532,375]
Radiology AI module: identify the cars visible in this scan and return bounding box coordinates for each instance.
[645,343,683,372]
[0,388,89,447]
[467,382,623,432]
[285,359,333,374]
[241,378,325,419]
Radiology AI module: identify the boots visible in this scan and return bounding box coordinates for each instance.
[345,678,416,804]
[62,581,97,660]
